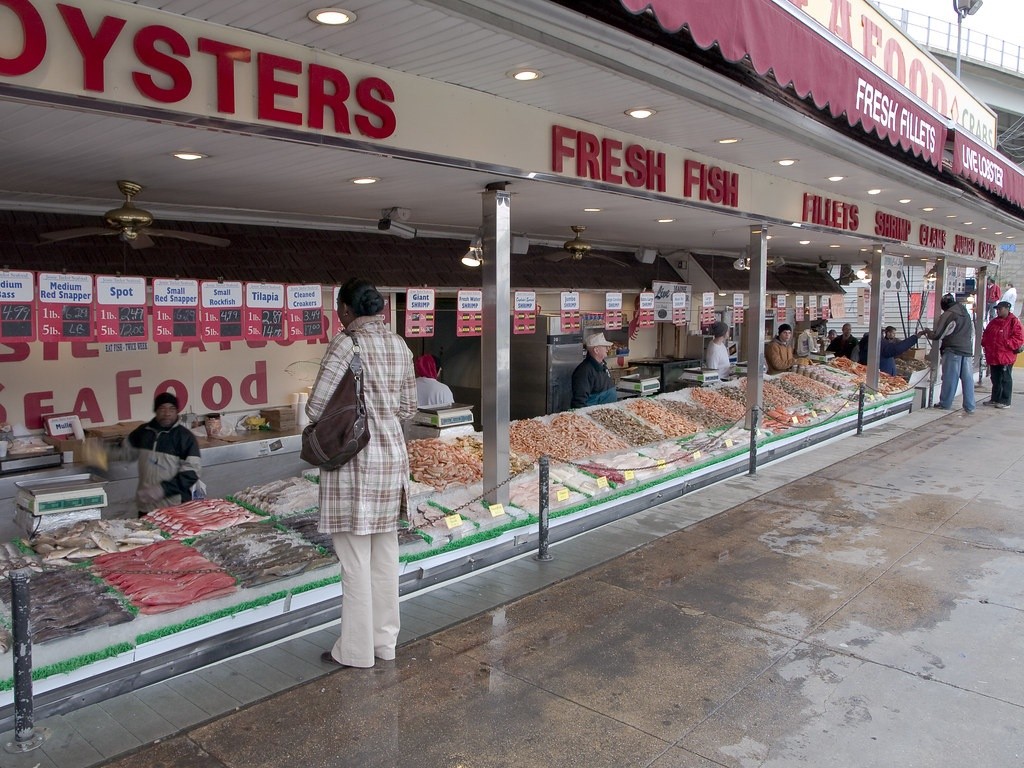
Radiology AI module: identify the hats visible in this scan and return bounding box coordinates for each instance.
[712,321,728,337]
[941,293,954,309]
[993,301,1011,309]
[778,324,791,336]
[583,332,614,347]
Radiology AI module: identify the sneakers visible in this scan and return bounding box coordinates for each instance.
[1010,317,1024,353]
[983,400,1011,409]
[934,402,946,410]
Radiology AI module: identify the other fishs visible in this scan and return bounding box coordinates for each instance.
[0,475,339,655]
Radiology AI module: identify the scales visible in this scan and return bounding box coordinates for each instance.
[810,351,836,362]
[616,373,661,393]
[414,401,474,428]
[13,473,109,516]
[736,361,768,375]
[678,367,721,383]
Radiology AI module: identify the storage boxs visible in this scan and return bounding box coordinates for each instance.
[42,431,99,465]
[904,349,925,360]
[259,408,296,422]
[265,419,297,432]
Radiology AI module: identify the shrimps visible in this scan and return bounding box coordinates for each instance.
[392,356,909,550]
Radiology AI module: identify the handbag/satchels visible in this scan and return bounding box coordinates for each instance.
[300,328,371,470]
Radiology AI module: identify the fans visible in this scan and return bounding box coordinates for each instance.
[767,247,808,275]
[39,179,231,249]
[540,225,630,269]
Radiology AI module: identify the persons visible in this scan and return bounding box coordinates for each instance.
[571,332,617,408]
[981,301,1024,409]
[305,276,418,670]
[415,354,455,407]
[926,293,976,413]
[985,277,1000,321]
[124,392,201,519]
[706,322,730,381]
[765,324,813,375]
[1001,282,1017,313]
[827,323,922,377]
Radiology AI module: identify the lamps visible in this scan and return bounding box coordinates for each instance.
[733,250,748,270]
[923,269,938,282]
[856,266,872,285]
[462,235,482,268]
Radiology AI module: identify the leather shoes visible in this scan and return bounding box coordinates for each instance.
[321,652,339,665]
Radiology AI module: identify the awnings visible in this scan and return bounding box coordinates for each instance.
[951,123,1024,209]
[619,0,950,173]
